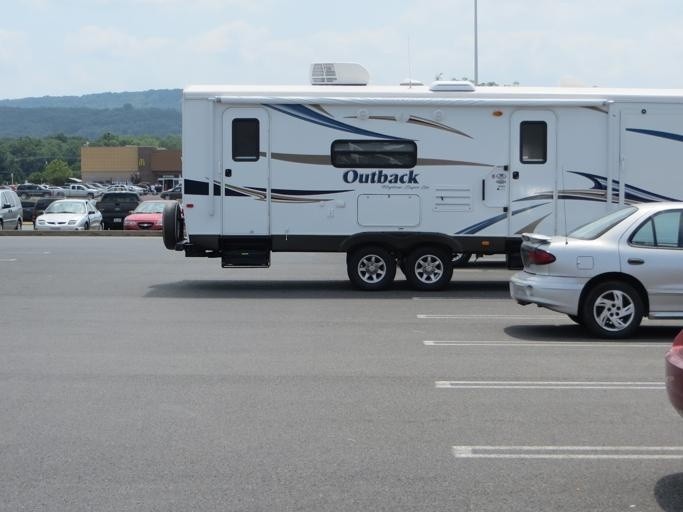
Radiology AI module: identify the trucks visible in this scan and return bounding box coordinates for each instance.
[164,60,683,291]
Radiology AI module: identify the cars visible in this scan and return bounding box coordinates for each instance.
[508,201,683,419]
[0,177,183,231]
